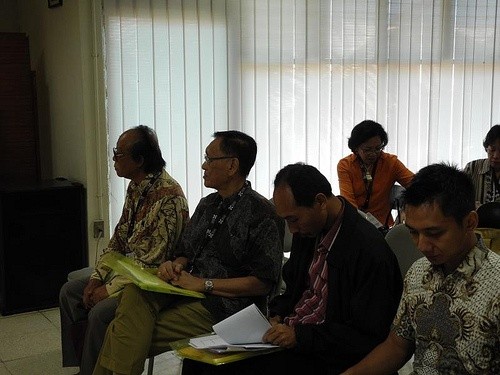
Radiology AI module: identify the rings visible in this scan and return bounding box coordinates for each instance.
[157,272,161,275]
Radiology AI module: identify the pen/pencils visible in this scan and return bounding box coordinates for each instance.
[266,295,270,320]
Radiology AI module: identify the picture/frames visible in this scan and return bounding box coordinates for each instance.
[47,0,64,9]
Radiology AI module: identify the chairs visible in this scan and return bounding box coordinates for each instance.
[383,223,426,280]
[268,198,293,290]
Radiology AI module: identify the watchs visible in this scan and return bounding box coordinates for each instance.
[205,280,214,293]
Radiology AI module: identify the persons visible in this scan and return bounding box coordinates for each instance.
[340,164,500,375]
[92,130,283,375]
[60,125,191,375]
[182,164,403,375]
[463,125,500,209]
[337,120,415,228]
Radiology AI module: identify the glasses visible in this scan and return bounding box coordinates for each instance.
[112,147,139,161]
[204,154,237,166]
[359,144,383,153]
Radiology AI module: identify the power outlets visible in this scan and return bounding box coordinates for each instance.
[92,219,105,239]
